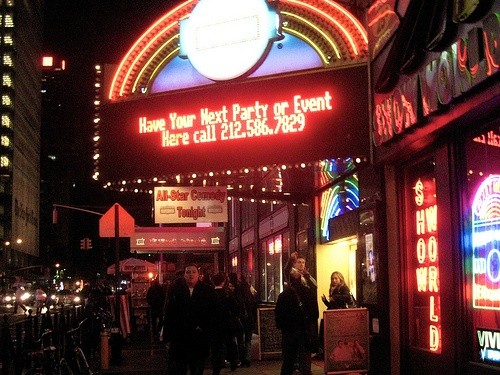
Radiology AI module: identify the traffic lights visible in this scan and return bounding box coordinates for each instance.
[86,238,93,250]
[80,238,86,250]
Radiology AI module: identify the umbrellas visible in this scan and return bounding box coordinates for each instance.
[107,258,157,287]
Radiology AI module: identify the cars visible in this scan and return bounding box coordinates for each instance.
[1,287,81,308]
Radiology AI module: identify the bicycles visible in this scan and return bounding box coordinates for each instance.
[26,319,95,375]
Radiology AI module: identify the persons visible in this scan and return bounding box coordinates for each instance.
[90,280,113,310]
[368,251,374,265]
[284,250,322,359]
[1,287,6,306]
[163,263,224,375]
[274,273,314,375]
[311,272,351,359]
[13,286,27,314]
[35,290,52,315]
[119,283,132,305]
[145,270,259,371]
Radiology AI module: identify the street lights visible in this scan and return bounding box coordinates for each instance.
[4,239,23,273]
